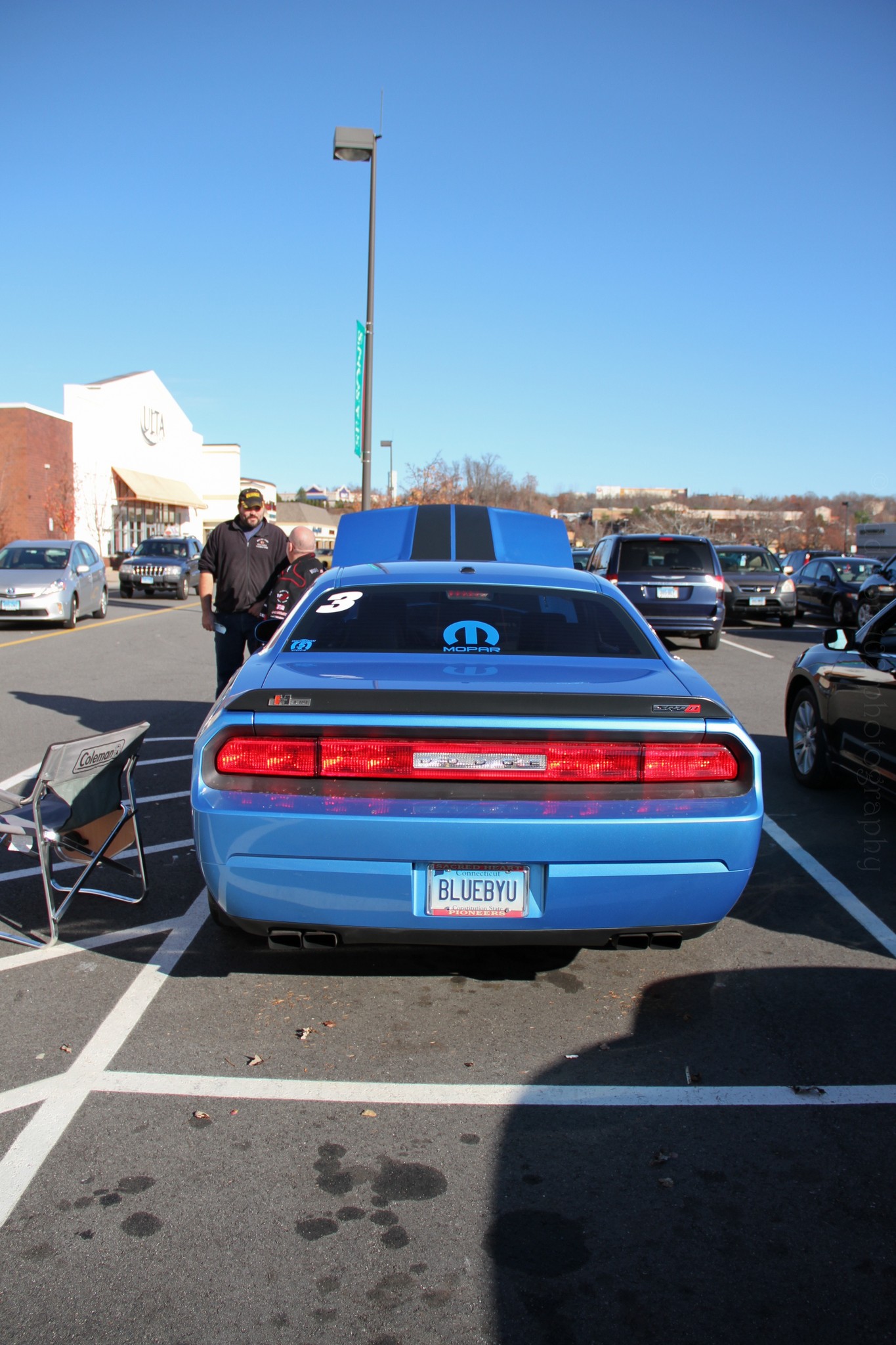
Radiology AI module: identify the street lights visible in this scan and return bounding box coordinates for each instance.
[331,124,378,511]
[380,440,394,508]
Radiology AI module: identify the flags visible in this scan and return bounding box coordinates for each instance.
[353,320,365,457]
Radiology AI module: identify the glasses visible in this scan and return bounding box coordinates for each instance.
[286,537,295,549]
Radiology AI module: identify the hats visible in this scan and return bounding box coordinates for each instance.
[239,488,263,508]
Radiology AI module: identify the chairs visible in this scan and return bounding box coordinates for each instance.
[631,549,648,568]
[0,720,151,949]
[745,555,762,568]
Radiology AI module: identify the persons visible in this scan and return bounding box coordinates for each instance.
[257,526,328,624]
[197,488,287,702]
[174,545,187,557]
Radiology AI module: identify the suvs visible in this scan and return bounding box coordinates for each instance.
[713,542,797,630]
[783,595,896,798]
[583,531,726,650]
[117,534,204,601]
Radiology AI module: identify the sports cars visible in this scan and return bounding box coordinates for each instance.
[188,503,767,976]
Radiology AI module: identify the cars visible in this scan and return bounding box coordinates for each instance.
[570,547,594,571]
[0,537,110,628]
[314,548,334,569]
[854,552,896,638]
[785,556,886,626]
[772,550,867,579]
